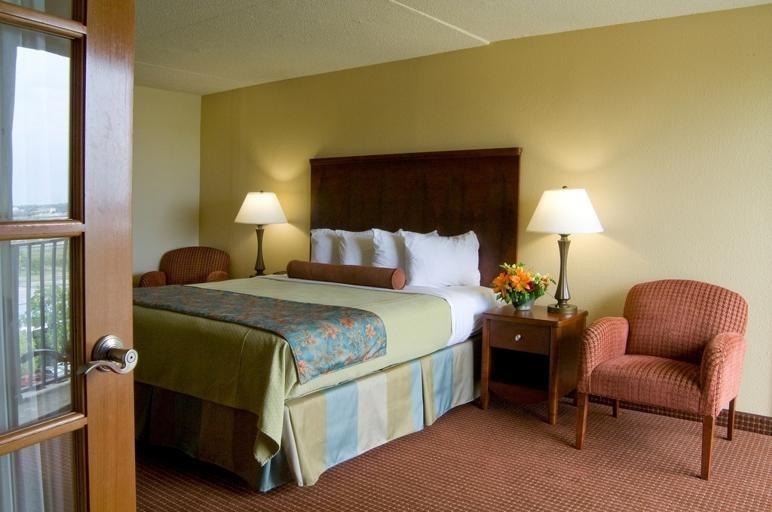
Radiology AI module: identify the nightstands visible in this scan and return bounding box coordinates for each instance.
[479,306,588,427]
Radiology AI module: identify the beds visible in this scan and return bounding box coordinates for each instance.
[131,144,523,493]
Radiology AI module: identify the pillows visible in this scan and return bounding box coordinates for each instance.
[287,227,483,290]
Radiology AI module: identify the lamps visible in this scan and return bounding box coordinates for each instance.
[524,188,606,315]
[233,189,288,278]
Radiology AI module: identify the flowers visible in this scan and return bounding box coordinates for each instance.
[492,260,557,304]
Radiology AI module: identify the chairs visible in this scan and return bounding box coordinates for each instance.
[138,246,230,288]
[573,278,751,483]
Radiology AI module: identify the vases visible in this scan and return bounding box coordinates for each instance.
[513,297,535,311]
